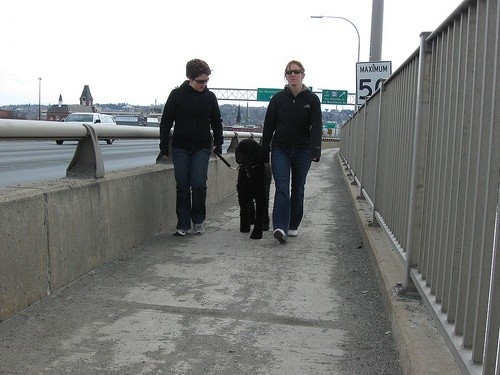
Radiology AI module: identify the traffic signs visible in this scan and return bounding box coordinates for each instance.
[322,89,348,105]
[257,87,283,101]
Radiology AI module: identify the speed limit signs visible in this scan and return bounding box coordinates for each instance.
[356,61,392,111]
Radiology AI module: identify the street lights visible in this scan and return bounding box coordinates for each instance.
[37,77,42,121]
[310,15,360,62]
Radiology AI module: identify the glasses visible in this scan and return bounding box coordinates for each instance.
[286,69,303,74]
[194,79,208,84]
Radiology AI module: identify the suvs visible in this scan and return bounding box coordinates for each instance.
[55,113,117,145]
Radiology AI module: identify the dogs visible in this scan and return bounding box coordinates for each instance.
[235,138,272,239]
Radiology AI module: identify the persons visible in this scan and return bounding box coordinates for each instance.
[160,58,224,236]
[263,61,322,245]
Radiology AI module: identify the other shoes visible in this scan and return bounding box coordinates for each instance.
[273,228,288,244]
[288,229,298,237]
[193,223,205,234]
[173,228,192,236]
